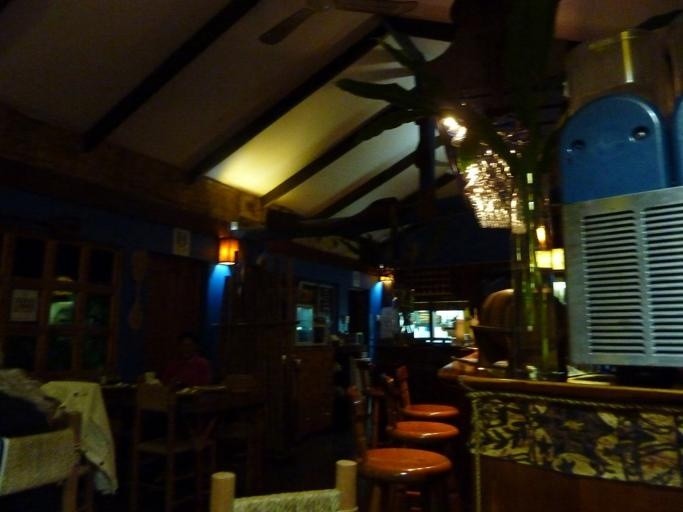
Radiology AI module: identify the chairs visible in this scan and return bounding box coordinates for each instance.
[210,457,359,512]
[130,374,217,512]
[2,379,119,512]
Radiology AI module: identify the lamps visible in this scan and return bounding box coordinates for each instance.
[217,237,239,265]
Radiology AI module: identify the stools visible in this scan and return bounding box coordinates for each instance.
[381,364,459,454]
[345,383,454,512]
[362,384,459,511]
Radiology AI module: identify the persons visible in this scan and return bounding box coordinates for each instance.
[161,333,212,387]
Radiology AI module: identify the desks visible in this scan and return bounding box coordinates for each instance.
[28,377,271,511]
[2,406,83,511]
